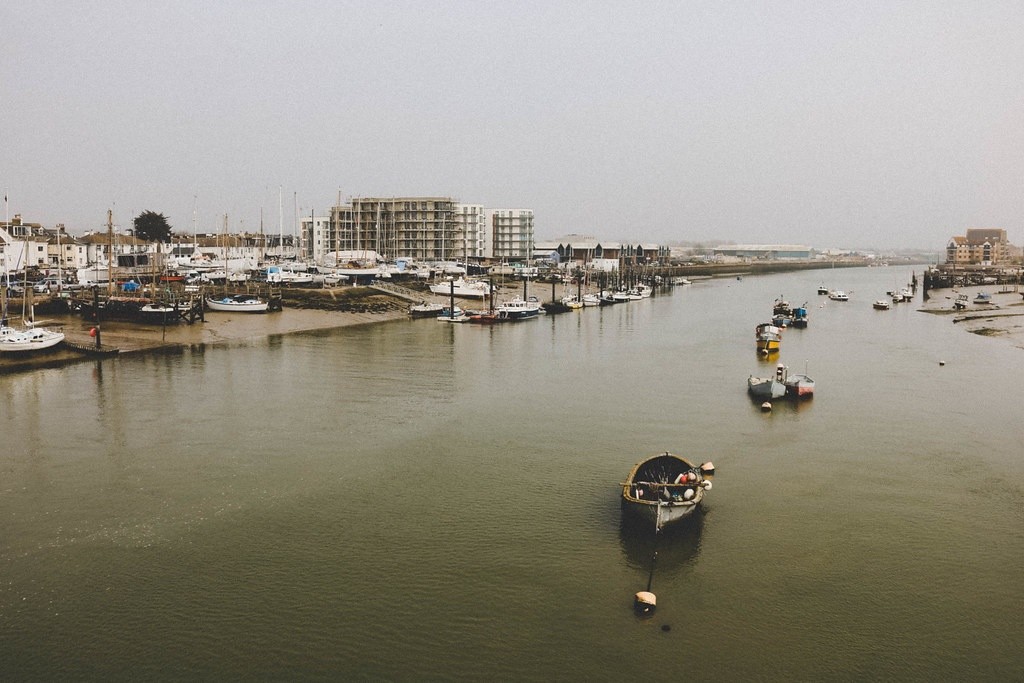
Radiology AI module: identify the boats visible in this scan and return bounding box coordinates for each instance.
[784,374,815,399]
[620,450,707,533]
[756,270,1004,356]
[0,239,697,360]
[748,375,787,402]
